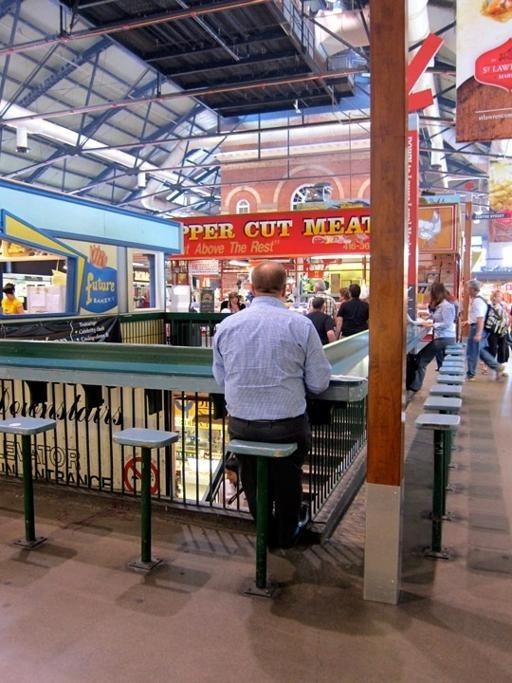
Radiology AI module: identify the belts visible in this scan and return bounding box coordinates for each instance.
[240,418,288,428]
[470,323,478,326]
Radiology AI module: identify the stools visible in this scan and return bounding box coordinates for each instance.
[436,373,466,470]
[444,348,467,414]
[446,343,468,400]
[429,383,463,494]
[419,394,463,523]
[0,417,57,551]
[413,412,461,559]
[438,365,466,452]
[111,427,180,572]
[444,353,467,427]
[224,438,300,600]
[441,359,467,438]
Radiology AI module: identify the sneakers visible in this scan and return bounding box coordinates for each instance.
[465,377,476,382]
[495,366,504,381]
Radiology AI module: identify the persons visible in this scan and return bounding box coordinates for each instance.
[212,260,323,552]
[464,280,509,382]
[220,292,246,312]
[337,287,350,312]
[480,289,509,375]
[490,290,496,306]
[408,282,456,392]
[238,292,250,307]
[307,279,336,318]
[1,283,24,315]
[218,456,248,509]
[305,297,336,346]
[335,284,370,341]
[503,290,512,351]
